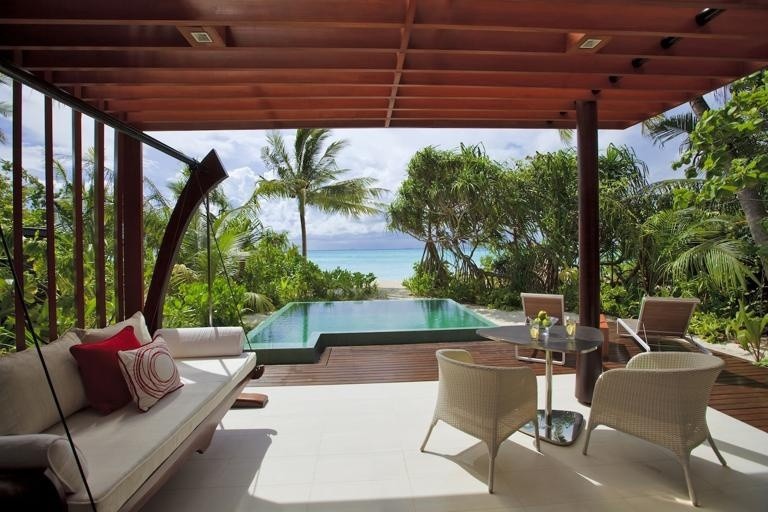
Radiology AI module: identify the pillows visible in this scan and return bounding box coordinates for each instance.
[70,309,154,347]
[112,333,187,414]
[0,327,89,436]
[65,322,145,417]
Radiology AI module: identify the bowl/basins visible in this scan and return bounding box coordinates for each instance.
[539,316,559,334]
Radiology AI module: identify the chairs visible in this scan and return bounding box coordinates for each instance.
[615,296,714,357]
[580,353,726,509]
[520,292,569,366]
[420,346,543,495]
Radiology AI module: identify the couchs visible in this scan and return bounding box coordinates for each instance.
[0,309,268,511]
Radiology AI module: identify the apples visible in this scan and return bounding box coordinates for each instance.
[533,318,542,326]
[538,310,547,319]
[542,319,552,328]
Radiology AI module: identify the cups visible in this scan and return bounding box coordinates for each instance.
[564,317,577,340]
[527,322,538,341]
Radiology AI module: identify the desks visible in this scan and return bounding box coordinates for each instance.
[598,312,610,359]
[475,323,606,449]
